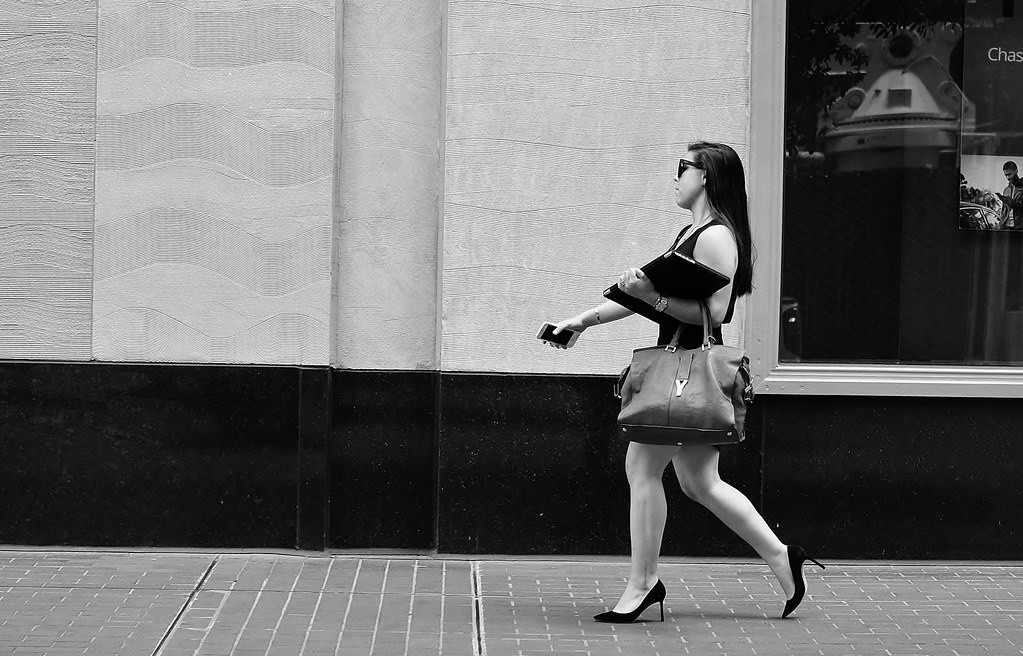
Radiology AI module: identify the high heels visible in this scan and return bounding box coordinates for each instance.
[782,544,825,618]
[594,577,666,624]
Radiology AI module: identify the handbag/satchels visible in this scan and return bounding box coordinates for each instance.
[613,301,754,446]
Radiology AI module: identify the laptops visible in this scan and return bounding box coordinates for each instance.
[603,250,731,327]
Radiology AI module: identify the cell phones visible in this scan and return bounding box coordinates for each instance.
[537,322,580,349]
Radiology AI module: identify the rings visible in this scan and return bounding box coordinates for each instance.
[620,280,625,286]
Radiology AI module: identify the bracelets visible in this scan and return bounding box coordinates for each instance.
[652,295,668,313]
[594,307,601,324]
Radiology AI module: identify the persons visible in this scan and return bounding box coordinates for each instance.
[541,141,825,623]
[995,161,1023,230]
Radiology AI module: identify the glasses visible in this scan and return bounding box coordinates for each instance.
[678,158,703,178]
